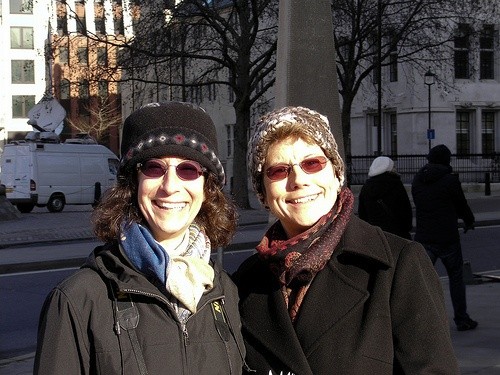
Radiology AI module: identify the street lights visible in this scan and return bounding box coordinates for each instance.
[423,65,436,151]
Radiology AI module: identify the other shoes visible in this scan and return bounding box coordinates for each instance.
[457,317,477,331]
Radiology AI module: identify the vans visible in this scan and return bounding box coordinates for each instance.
[0,138,121,215]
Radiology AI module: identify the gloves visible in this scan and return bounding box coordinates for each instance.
[463,224,475,233]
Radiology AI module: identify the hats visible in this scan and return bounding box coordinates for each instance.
[117,101,225,190]
[368,156,394,177]
[426,144,451,164]
[248,107,344,209]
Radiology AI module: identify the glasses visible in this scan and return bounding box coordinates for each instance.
[263,156,330,181]
[137,158,209,180]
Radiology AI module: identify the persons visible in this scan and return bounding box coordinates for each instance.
[413,144,479,332]
[357,155,413,241]
[33,100,247,375]
[227,105,458,375]
